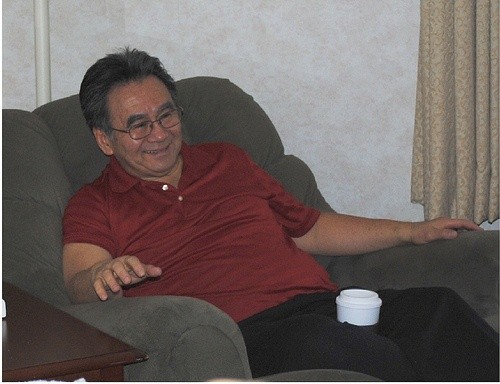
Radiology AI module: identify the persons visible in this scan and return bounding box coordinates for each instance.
[61,46,500,382]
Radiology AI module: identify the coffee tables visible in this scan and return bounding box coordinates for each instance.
[3,281,149,381]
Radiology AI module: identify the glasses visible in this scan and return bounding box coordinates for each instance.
[107,104,184,140]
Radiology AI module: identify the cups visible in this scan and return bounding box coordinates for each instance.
[336,289,382,326]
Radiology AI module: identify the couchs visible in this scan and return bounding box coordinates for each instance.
[3,76,499,381]
